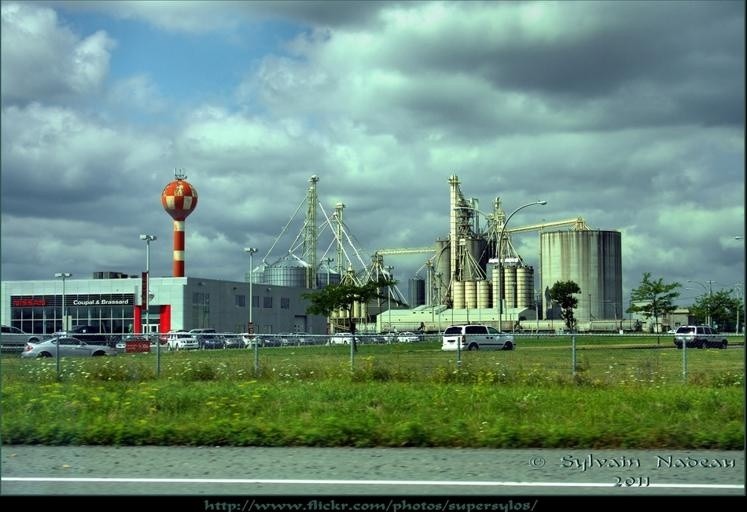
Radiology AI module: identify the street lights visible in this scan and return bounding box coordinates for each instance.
[734,236,743,240]
[384,264,396,327]
[243,248,257,333]
[685,287,707,324]
[55,271,71,334]
[687,280,710,325]
[452,200,548,333]
[139,234,156,340]
[322,258,335,323]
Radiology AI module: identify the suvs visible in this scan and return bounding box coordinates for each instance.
[673,325,727,350]
[442,325,516,353]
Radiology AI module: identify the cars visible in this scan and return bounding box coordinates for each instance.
[1,324,422,357]
